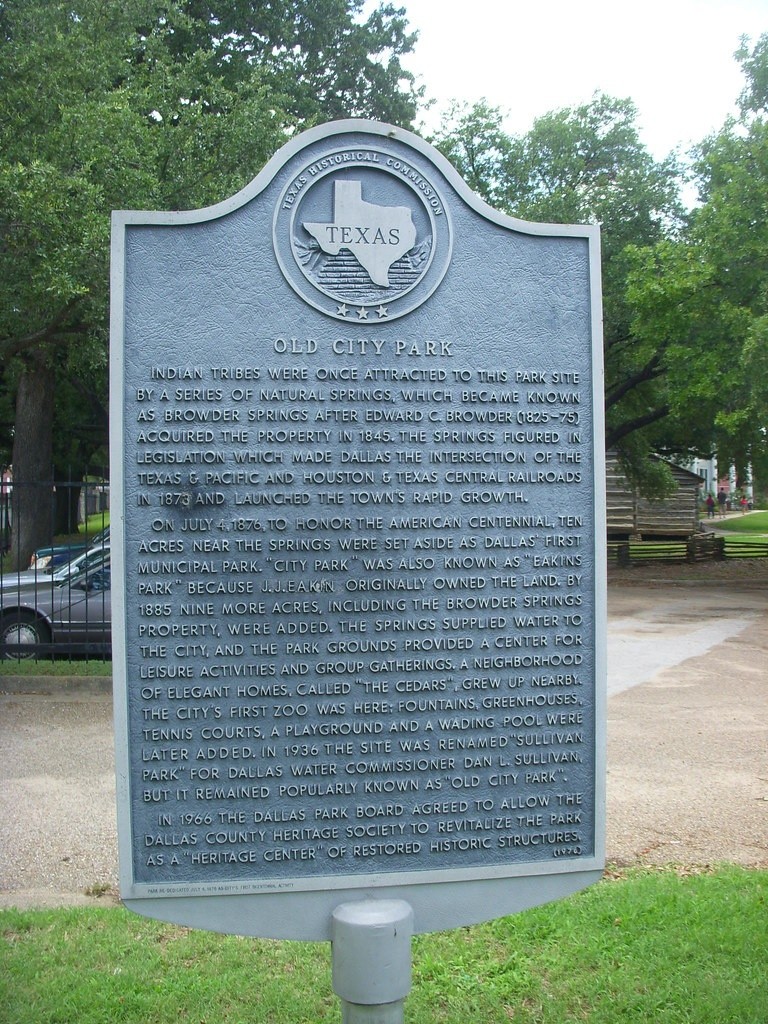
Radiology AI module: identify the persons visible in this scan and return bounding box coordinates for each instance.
[717,488,727,519]
[740,495,747,515]
[706,494,715,518]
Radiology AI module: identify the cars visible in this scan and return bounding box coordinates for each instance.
[0,524,110,660]
